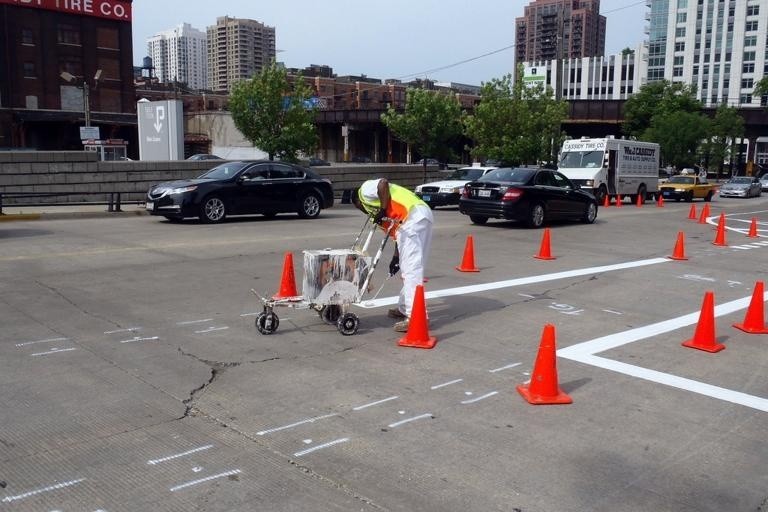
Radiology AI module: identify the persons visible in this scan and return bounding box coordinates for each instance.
[350,177,433,332]
[671,165,676,175]
[665,163,671,177]
[693,162,707,184]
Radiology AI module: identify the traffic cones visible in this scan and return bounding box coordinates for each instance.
[533,228,557,262]
[732,282,768,334]
[456,236,480,274]
[666,231,688,263]
[691,204,760,246]
[271,253,301,306]
[515,323,571,405]
[681,291,726,353]
[397,284,438,351]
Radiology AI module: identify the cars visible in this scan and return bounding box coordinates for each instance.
[145,160,335,222]
[187,153,225,161]
[305,155,332,166]
[97,152,132,161]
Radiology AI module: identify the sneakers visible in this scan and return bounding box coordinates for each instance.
[393,318,430,332]
[387,308,407,320]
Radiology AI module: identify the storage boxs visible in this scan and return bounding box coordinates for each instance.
[301,248,362,302]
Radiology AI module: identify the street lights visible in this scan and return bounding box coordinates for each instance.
[60,67,103,127]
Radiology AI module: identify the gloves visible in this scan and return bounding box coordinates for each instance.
[389,255,400,276]
[372,208,387,225]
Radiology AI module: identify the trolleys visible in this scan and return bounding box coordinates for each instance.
[242,208,396,335]
